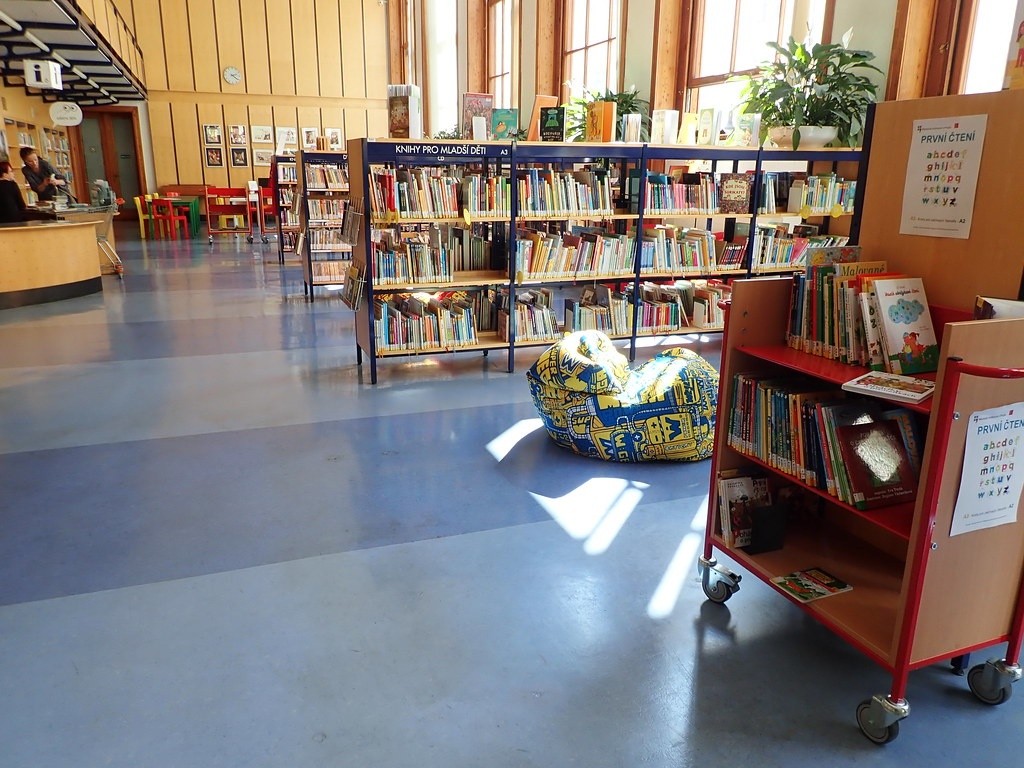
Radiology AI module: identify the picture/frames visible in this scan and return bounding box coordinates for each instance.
[227,125,247,146]
[325,128,342,148]
[203,124,223,146]
[276,127,297,145]
[230,147,249,168]
[253,150,274,167]
[276,148,295,155]
[251,126,273,144]
[302,127,319,148]
[205,147,224,168]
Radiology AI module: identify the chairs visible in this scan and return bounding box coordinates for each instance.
[139,195,168,237]
[153,192,180,228]
[145,195,170,233]
[217,198,245,238]
[133,197,165,239]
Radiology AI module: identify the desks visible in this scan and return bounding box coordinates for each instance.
[230,198,248,238]
[145,196,201,240]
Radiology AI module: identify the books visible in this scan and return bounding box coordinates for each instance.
[16,132,119,214]
[268,85,938,601]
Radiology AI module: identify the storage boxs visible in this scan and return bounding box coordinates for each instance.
[387,96,421,139]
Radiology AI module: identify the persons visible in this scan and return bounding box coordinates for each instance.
[0,161,26,224]
[19,147,78,204]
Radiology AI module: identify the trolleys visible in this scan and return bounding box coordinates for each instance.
[54,198,124,279]
[700,274,1023,744]
[204,186,251,244]
[258,186,277,243]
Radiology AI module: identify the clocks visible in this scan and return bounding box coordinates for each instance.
[223,66,242,85]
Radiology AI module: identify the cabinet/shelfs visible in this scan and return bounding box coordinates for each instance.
[695,275,1024,744]
[0,113,76,205]
[346,138,862,385]
[257,177,277,244]
[848,88,1024,306]
[204,185,254,246]
[295,149,640,302]
[271,155,350,266]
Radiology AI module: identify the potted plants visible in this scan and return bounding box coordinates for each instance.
[725,20,886,152]
